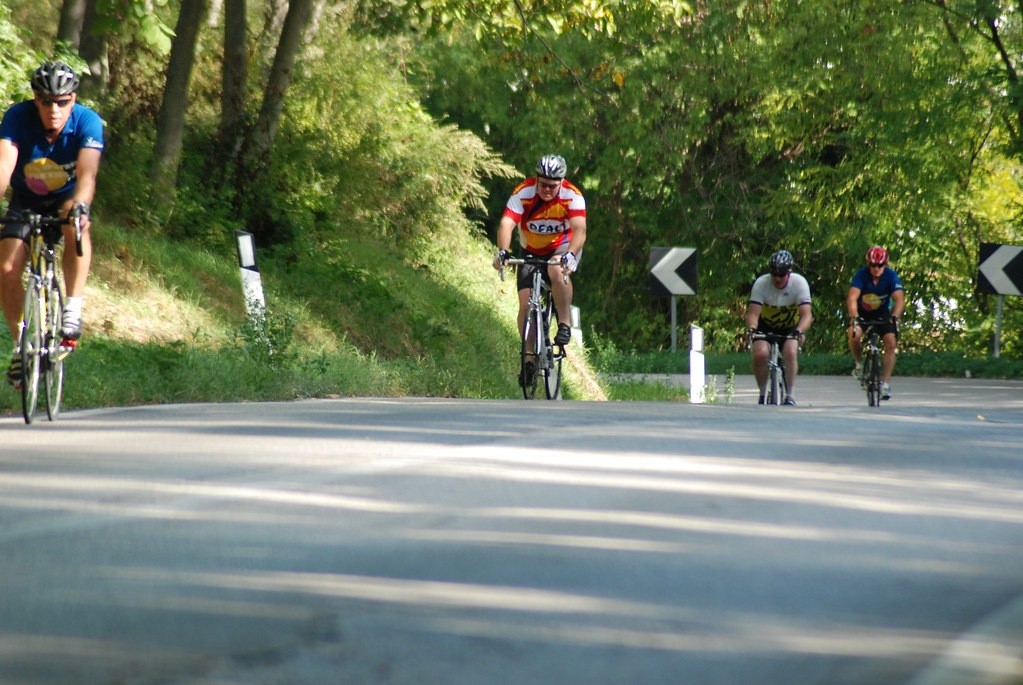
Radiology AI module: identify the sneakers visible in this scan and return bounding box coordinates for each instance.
[518,363,534,386]
[555,323,571,344]
[60,309,82,339]
[7,354,25,385]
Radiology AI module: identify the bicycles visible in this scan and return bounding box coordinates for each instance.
[746,327,804,405]
[851,314,899,407]
[0,201,88,425]
[497,250,567,401]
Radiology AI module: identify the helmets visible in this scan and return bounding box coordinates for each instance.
[769,250,794,272]
[536,154,567,178]
[31,61,80,97]
[865,246,889,264]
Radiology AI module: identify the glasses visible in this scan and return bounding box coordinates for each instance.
[540,182,561,189]
[770,272,787,277]
[42,99,71,108]
[872,264,883,268]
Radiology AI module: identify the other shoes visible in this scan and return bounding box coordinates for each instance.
[852,366,863,378]
[880,387,890,400]
[759,396,764,404]
[784,399,795,406]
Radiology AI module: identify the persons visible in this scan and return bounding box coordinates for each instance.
[848,246,905,397]
[492,154,586,387]
[0,60,104,389]
[747,250,813,405]
